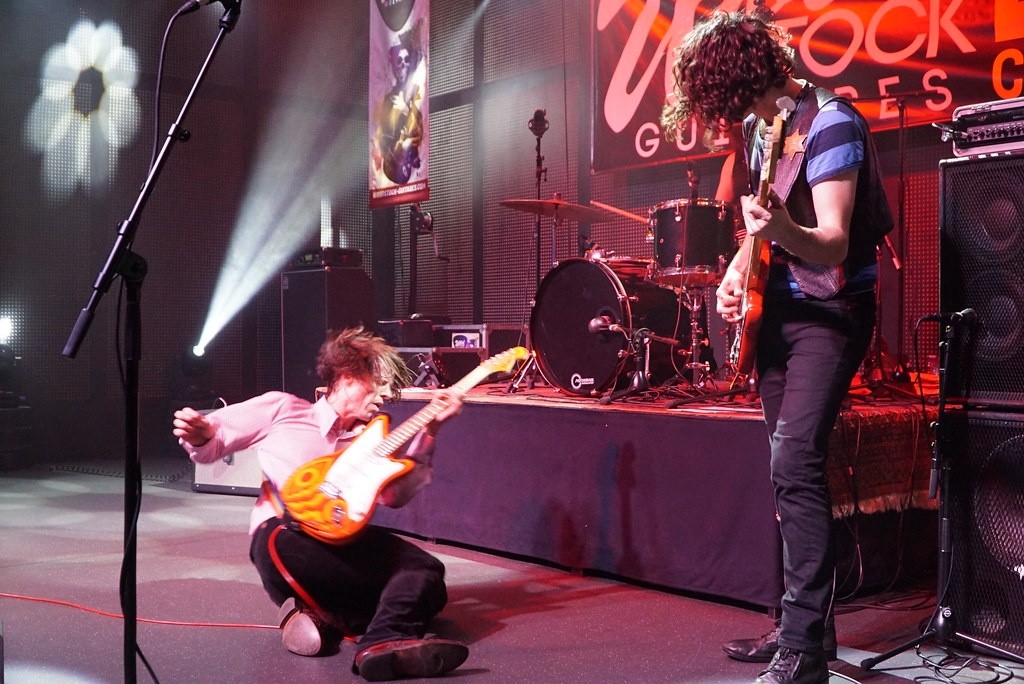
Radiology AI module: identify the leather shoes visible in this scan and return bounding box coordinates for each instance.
[355,637,470,682]
[721,617,837,661]
[752,645,829,684]
[277,598,328,656]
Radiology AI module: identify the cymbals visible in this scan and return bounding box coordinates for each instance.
[497,196,615,226]
[592,255,652,269]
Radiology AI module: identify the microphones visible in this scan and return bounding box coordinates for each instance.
[581,235,602,250]
[686,161,696,187]
[177,0,218,16]
[532,110,545,139]
[589,316,614,334]
[924,308,978,326]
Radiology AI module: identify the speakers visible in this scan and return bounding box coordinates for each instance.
[281,266,366,403]
[935,147,1024,660]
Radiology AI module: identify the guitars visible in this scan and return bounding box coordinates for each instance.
[727,111,788,376]
[274,343,532,545]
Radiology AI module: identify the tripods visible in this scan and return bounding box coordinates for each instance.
[846,234,936,407]
[600,287,722,405]
[860,323,1024,670]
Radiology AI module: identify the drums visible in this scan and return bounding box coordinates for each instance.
[528,253,698,403]
[644,196,739,289]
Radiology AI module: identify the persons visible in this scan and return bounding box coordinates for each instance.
[659,6,894,683]
[170,322,471,680]
